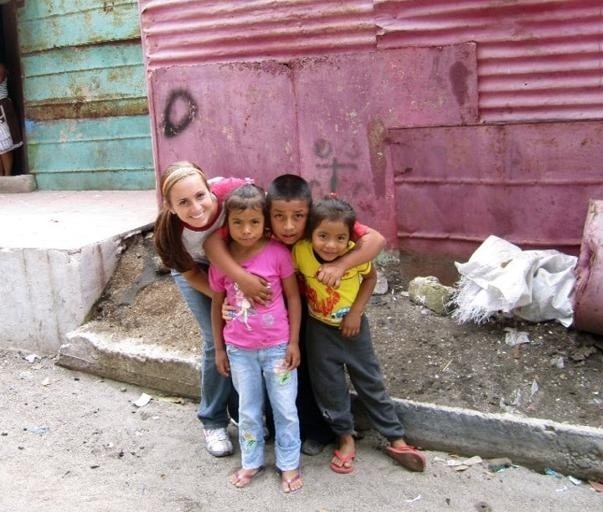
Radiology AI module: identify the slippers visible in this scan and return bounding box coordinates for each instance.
[234,466,264,485]
[330,448,355,473]
[274,464,302,494]
[383,446,425,472]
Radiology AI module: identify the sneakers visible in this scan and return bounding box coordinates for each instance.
[229,418,270,439]
[202,426,233,457]
[301,437,325,455]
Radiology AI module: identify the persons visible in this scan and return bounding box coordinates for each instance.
[199,171,388,458]
[0,63,23,177]
[206,182,307,495]
[288,190,426,477]
[155,157,253,457]
